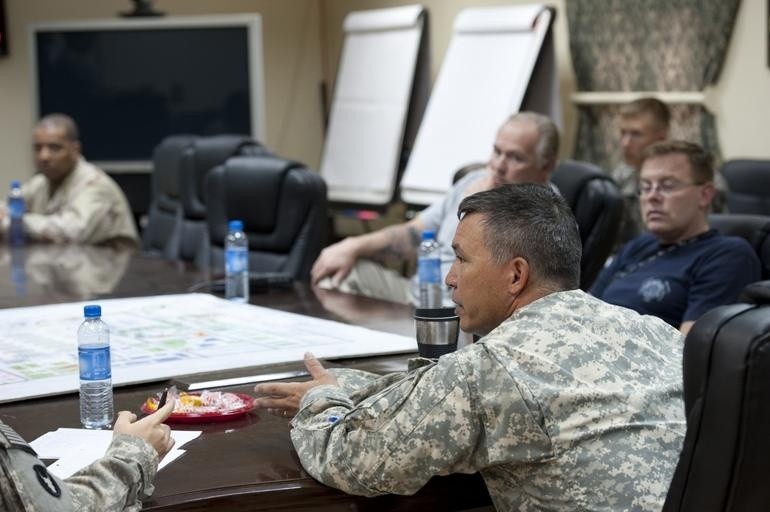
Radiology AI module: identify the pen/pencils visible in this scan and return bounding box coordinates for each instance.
[156,388,168,411]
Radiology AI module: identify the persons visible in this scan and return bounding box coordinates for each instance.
[309,110,580,310]
[1,112,142,251]
[588,138,746,337]
[253,181,684,512]
[1,396,180,512]
[615,99,731,242]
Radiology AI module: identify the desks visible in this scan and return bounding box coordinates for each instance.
[1,245,476,512]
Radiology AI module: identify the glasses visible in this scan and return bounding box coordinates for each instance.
[632,176,708,199]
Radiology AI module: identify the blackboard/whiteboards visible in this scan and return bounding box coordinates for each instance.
[399,3,555,209]
[317,3,426,207]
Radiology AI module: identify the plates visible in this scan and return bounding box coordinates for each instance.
[168,413,261,436]
[139,390,256,423]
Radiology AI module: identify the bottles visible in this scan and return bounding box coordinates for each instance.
[8,242,29,294]
[76,305,115,430]
[416,231,444,309]
[224,220,251,304]
[7,181,25,245]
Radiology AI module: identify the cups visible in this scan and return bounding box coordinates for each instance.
[412,307,460,359]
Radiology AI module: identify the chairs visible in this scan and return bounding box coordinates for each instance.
[168,134,267,262]
[197,153,326,282]
[142,137,187,251]
[660,279,769,511]
[719,159,770,214]
[452,160,624,294]
[706,212,769,274]
